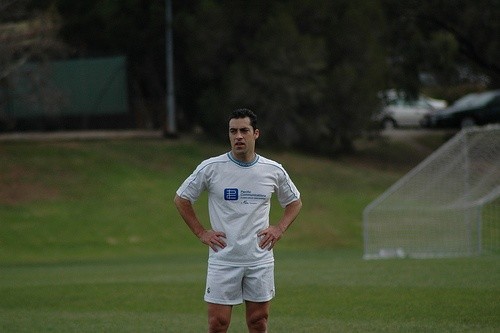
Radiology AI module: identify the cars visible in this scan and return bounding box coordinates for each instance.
[420,89,500,132]
[371,86,447,131]
